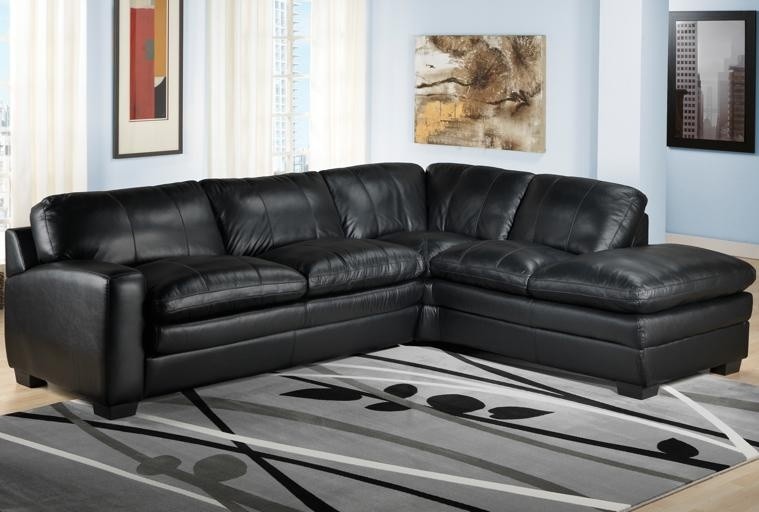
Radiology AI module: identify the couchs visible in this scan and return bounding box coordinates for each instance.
[5,162,756,420]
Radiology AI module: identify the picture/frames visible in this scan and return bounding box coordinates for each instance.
[113,0,184,159]
[667,11,757,153]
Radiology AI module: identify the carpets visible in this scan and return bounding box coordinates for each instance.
[1,346,759,511]
